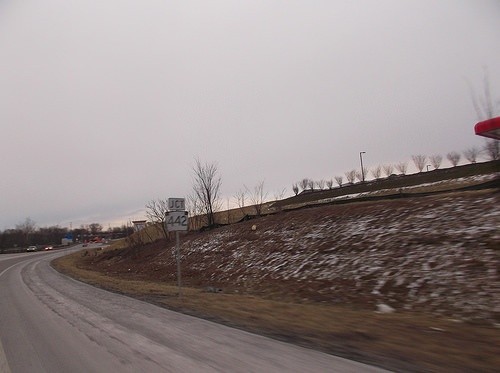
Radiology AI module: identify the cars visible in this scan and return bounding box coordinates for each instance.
[27,245,38,252]
[45,244,54,250]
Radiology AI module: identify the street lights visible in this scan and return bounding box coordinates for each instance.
[360,151,366,183]
